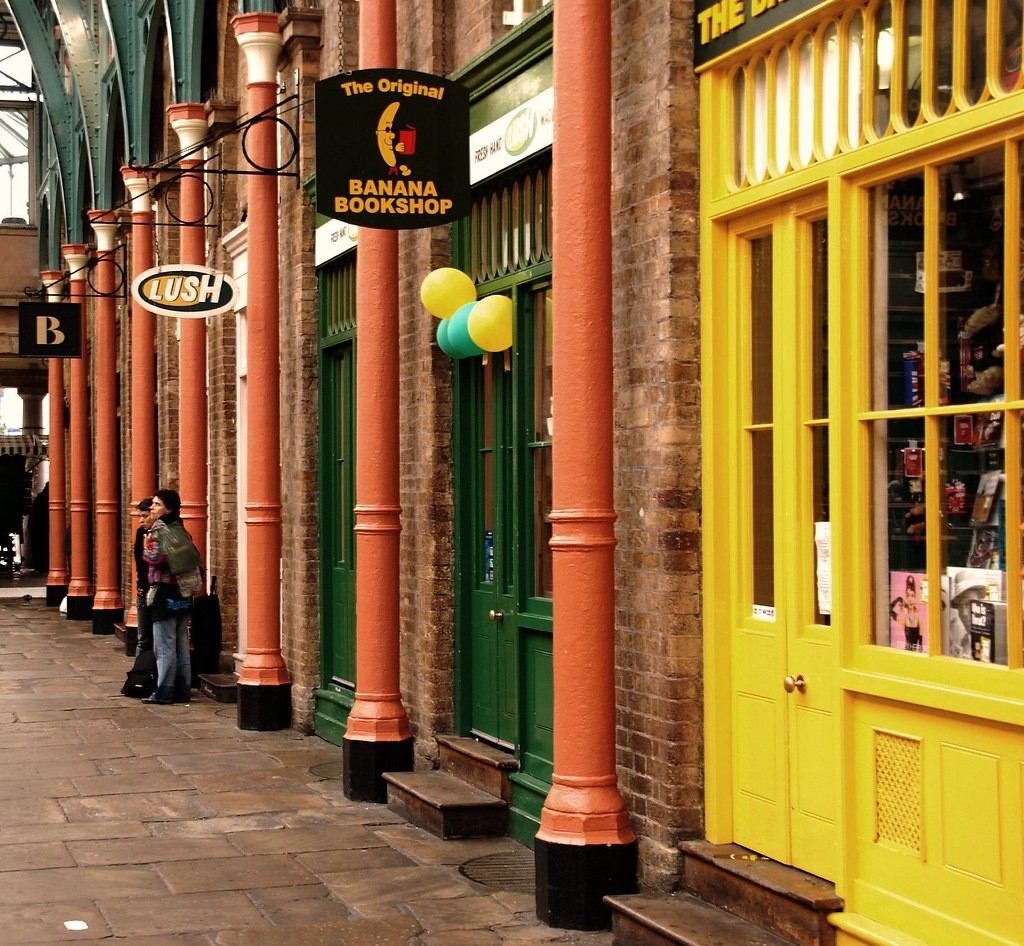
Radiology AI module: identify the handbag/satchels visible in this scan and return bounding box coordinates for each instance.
[157,524,203,576]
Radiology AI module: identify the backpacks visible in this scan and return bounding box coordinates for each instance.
[126,649,158,698]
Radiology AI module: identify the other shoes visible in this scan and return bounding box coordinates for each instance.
[121,685,127,695]
[142,695,187,704]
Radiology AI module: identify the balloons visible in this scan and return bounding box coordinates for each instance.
[421,267,514,359]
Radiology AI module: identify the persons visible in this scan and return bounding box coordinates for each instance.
[889,575,923,653]
[970,541,994,568]
[970,487,994,522]
[120,498,222,699]
[948,569,989,661]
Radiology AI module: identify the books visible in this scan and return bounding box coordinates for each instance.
[886,469,1007,664]
